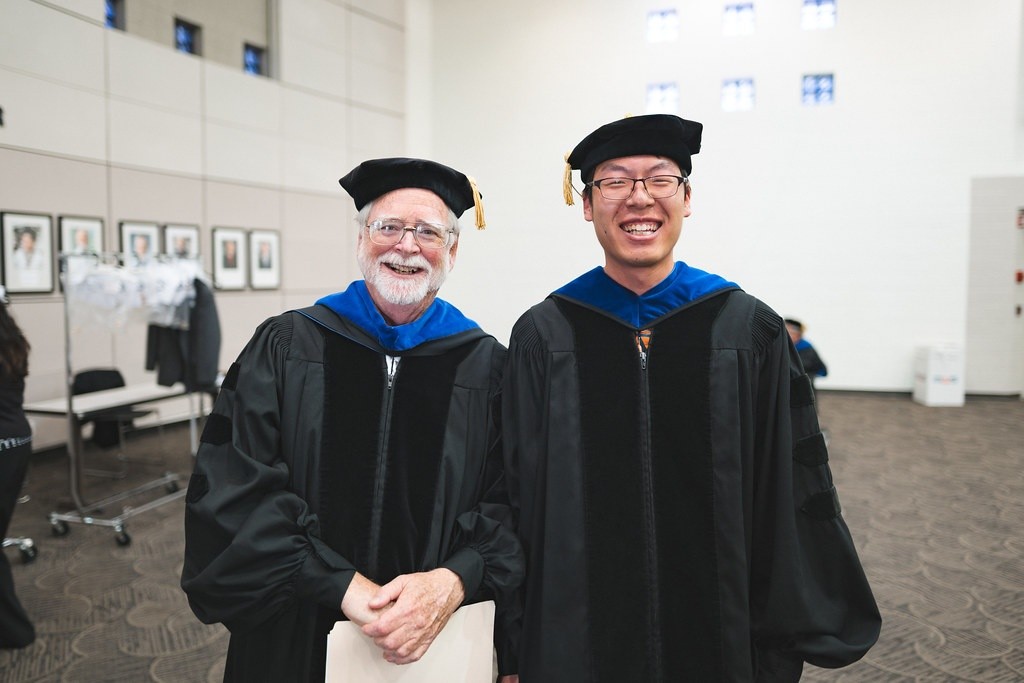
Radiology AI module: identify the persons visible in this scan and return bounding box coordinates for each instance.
[181,156,527,683]
[11,227,274,284]
[0,298,36,650]
[494,114,882,683]
[784,319,827,399]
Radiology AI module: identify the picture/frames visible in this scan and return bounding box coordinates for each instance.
[211,227,247,291]
[119,222,160,266]
[163,224,199,260]
[248,229,281,291]
[1,212,53,295]
[58,216,105,291]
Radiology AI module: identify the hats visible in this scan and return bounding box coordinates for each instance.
[563,114,702,205]
[339,162,485,230]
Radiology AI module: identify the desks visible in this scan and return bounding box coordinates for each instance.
[22,377,186,512]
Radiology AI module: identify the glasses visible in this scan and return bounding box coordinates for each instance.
[586,175,687,200]
[366,219,455,249]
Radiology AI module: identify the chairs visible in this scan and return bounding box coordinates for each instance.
[73,370,162,480]
